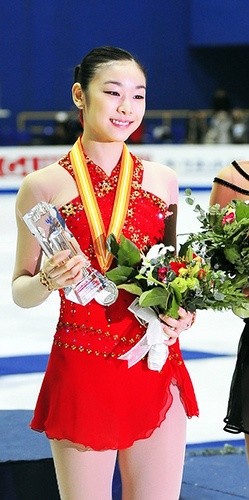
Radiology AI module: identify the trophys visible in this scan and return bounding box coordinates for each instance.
[22,201,102,307]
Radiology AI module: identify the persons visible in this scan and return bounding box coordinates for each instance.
[9,45,198,500]
[207,162,249,463]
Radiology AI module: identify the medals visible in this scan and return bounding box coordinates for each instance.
[94,277,119,306]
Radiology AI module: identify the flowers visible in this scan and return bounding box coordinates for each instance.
[103,187,249,373]
